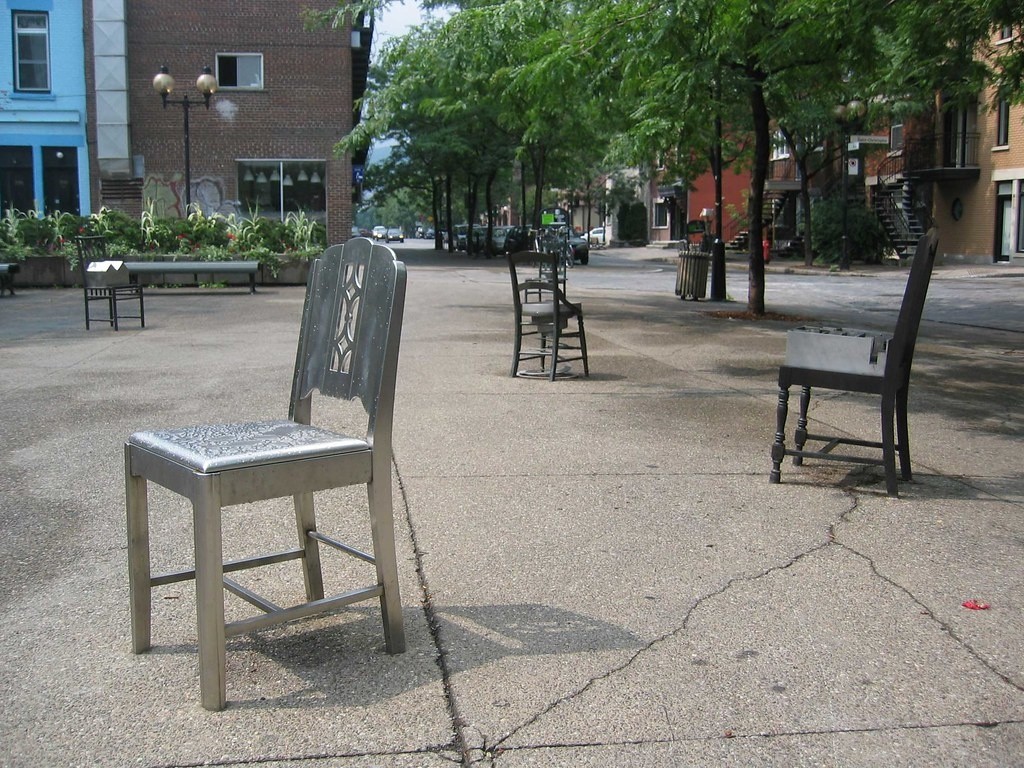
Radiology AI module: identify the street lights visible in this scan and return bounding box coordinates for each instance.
[152,64,217,217]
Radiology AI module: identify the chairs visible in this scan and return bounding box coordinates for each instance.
[525,233,567,303]
[76,236,146,331]
[767,228,940,496]
[505,250,589,382]
[121,236,407,711]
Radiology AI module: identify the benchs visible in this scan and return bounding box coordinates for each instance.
[125,260,261,293]
[0,264,21,297]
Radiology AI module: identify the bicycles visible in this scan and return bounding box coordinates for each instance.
[528,226,575,270]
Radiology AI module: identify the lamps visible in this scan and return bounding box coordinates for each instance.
[243,167,254,181]
[296,170,309,181]
[270,170,280,181]
[311,172,321,183]
[256,172,267,183]
[283,175,293,186]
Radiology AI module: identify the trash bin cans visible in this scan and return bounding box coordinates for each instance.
[675,241,713,300]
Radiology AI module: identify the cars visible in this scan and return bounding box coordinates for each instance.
[423,223,589,265]
[371,225,404,243]
[580,227,606,244]
